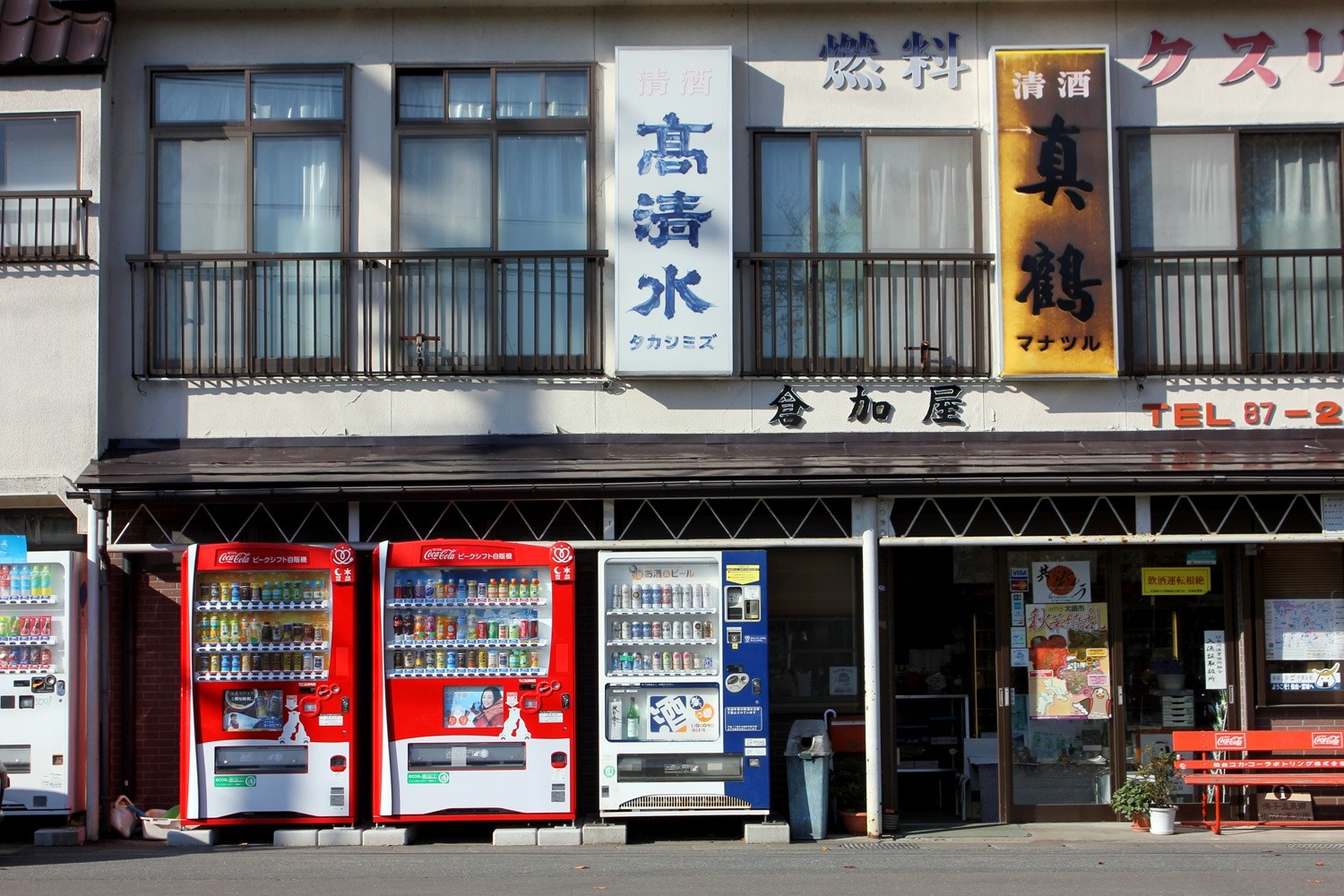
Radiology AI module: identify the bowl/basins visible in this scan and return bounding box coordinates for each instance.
[1157,674,1185,690]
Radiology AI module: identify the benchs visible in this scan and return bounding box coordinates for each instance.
[1170,729,1343,836]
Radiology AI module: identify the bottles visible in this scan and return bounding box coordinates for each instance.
[200,580,330,673]
[394,576,542,675]
[268,692,280,717]
[0,564,54,669]
[627,697,639,739]
[255,691,268,719]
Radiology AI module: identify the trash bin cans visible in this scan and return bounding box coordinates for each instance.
[783,720,832,839]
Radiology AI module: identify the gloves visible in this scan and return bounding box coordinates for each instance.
[471,705,484,720]
[469,703,482,710]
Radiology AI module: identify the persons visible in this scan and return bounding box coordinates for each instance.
[230,714,240,728]
[470,686,503,727]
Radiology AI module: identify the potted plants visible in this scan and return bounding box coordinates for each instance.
[1110,777,1160,833]
[1135,742,1188,837]
